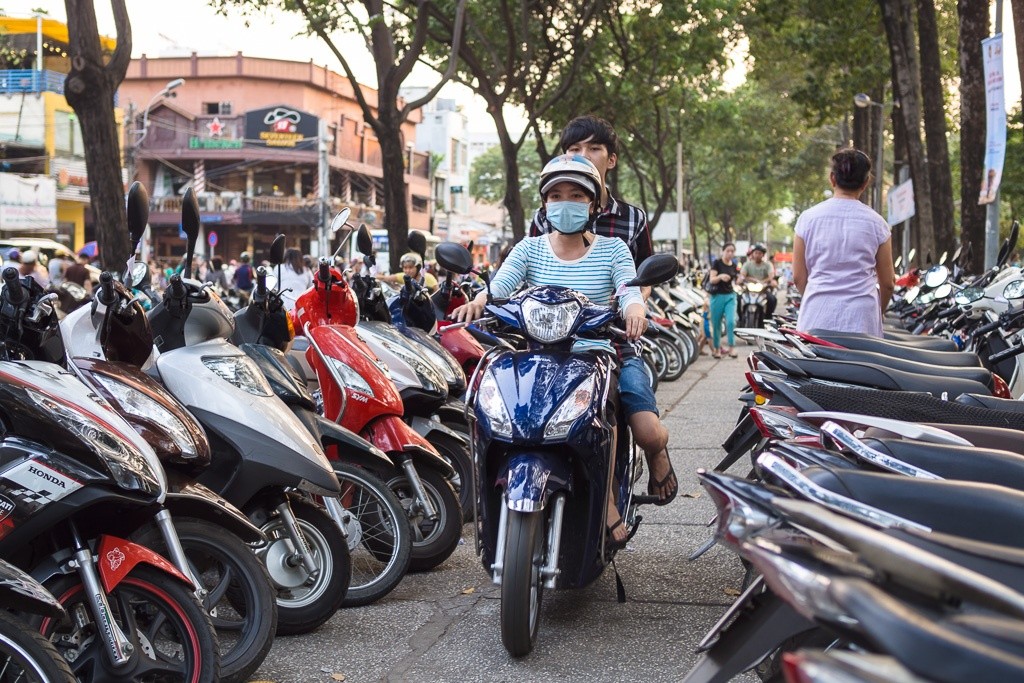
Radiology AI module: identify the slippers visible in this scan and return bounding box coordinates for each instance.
[645,445,678,505]
[606,517,628,547]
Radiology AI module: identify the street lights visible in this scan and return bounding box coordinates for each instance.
[668,103,688,263]
[128,78,184,196]
[852,93,900,215]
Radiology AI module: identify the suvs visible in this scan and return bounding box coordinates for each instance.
[0,238,103,290]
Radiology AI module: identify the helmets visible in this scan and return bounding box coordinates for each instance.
[539,154,602,202]
[240,251,251,261]
[399,252,422,269]
[54,249,66,256]
[752,242,767,253]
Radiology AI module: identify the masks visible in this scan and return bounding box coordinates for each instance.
[544,201,593,233]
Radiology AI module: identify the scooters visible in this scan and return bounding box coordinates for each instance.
[677,219,1024,683]
[0,182,741,683]
[436,242,680,657]
[734,276,781,345]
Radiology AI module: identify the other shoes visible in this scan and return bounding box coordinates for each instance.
[712,352,721,358]
[728,353,738,358]
[699,350,709,355]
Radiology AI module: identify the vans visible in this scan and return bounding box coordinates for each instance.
[351,228,434,275]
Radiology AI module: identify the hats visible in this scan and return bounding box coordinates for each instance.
[22,250,35,263]
[8,247,19,259]
[541,173,596,199]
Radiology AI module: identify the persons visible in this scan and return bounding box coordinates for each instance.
[377,253,439,294]
[452,153,648,544]
[0,247,172,291]
[708,242,740,358]
[177,247,379,311]
[736,243,778,320]
[792,148,896,337]
[528,116,679,507]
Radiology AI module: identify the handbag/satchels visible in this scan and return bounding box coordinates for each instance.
[701,259,721,296]
